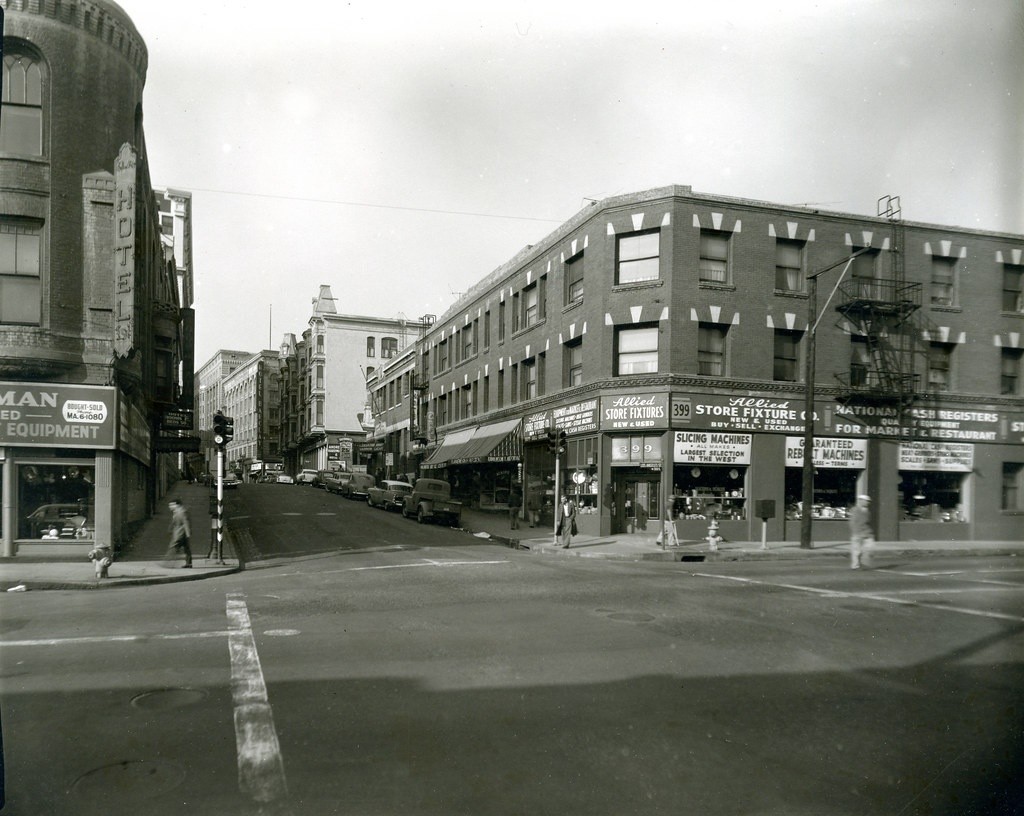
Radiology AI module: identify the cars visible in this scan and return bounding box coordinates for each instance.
[342,473,375,498]
[277,473,294,483]
[366,480,413,511]
[402,478,462,523]
[312,469,335,487]
[325,472,350,493]
[295,469,318,485]
[26,503,94,537]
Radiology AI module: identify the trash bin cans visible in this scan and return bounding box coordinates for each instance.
[210,495,217,514]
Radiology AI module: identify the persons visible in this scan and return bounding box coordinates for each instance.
[555,495,579,549]
[187,463,197,484]
[159,499,192,568]
[527,486,542,528]
[508,490,522,530]
[252,478,262,485]
[849,496,875,570]
[656,495,680,547]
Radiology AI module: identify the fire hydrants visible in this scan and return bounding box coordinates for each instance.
[702,520,723,550]
[86,543,114,578]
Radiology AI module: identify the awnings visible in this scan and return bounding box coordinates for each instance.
[155,409,202,455]
[419,417,524,471]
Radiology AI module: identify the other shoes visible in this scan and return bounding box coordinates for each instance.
[511,528,516,530]
[852,567,863,571]
[656,542,662,547]
[529,525,534,529]
[563,546,570,549]
[182,564,193,569]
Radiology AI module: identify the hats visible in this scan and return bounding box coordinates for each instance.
[858,494,872,502]
[668,494,677,499]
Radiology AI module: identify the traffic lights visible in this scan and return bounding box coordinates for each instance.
[546,430,556,454]
[224,414,234,445]
[213,414,224,445]
[557,430,567,454]
